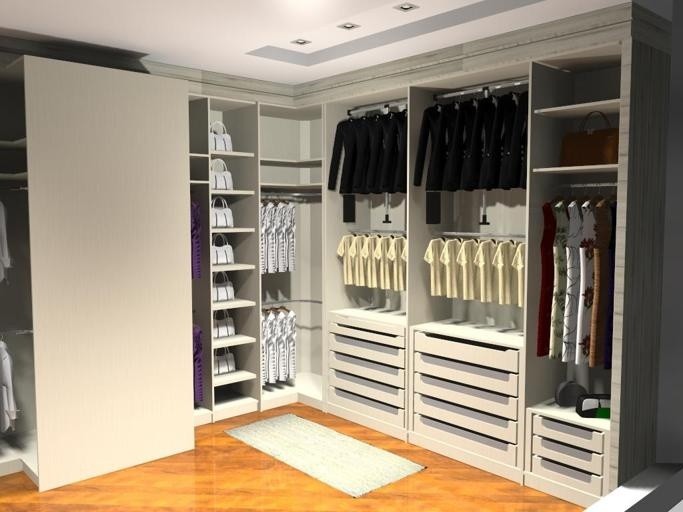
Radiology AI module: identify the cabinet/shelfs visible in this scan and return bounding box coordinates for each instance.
[209,41,323,425]
[323,42,669,506]
[0,41,208,494]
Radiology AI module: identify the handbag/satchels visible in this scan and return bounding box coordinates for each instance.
[209,121,233,151]
[213,271,236,302]
[213,348,235,375]
[210,158,234,190]
[212,234,235,265]
[555,381,610,419]
[561,110,619,165]
[213,310,236,338]
[211,197,234,228]
